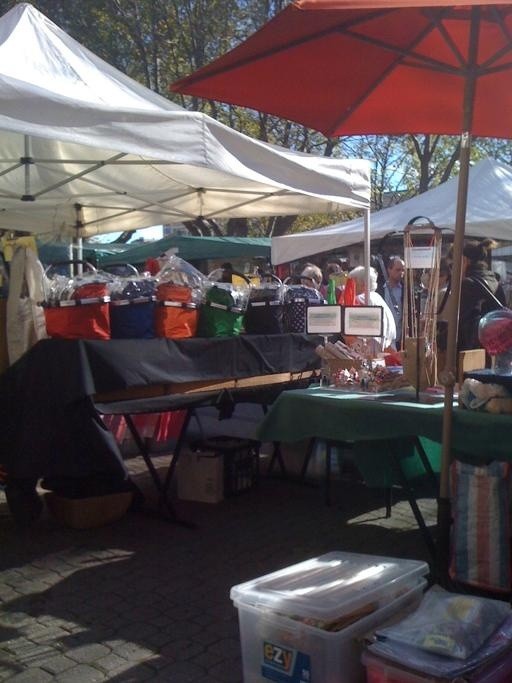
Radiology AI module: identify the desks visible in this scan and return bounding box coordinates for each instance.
[95,375,511,596]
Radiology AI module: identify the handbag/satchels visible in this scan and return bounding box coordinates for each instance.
[178,448,224,504]
[39,262,325,341]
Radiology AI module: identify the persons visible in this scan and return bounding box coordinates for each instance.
[217,262,249,283]
[301,235,507,349]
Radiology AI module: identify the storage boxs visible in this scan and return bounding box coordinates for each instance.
[228,550,512,683]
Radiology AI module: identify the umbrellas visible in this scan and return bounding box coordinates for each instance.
[170,0,512,588]
